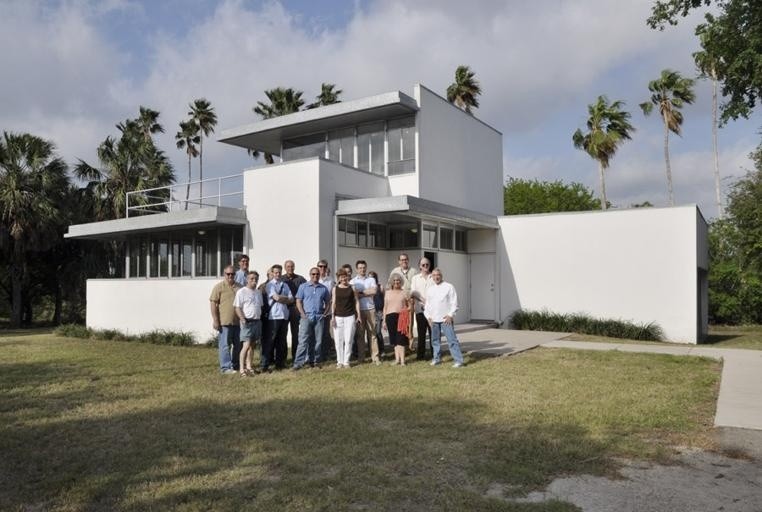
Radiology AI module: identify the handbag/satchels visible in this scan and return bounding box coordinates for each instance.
[261,303,271,322]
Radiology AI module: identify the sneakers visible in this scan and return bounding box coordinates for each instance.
[222,369,237,374]
[241,355,330,378]
[336,347,416,370]
[452,364,461,368]
[429,360,438,365]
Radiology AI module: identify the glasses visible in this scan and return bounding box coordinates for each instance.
[226,273,234,275]
[318,265,327,268]
[312,273,319,275]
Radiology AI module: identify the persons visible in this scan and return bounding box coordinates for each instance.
[208,253,467,378]
